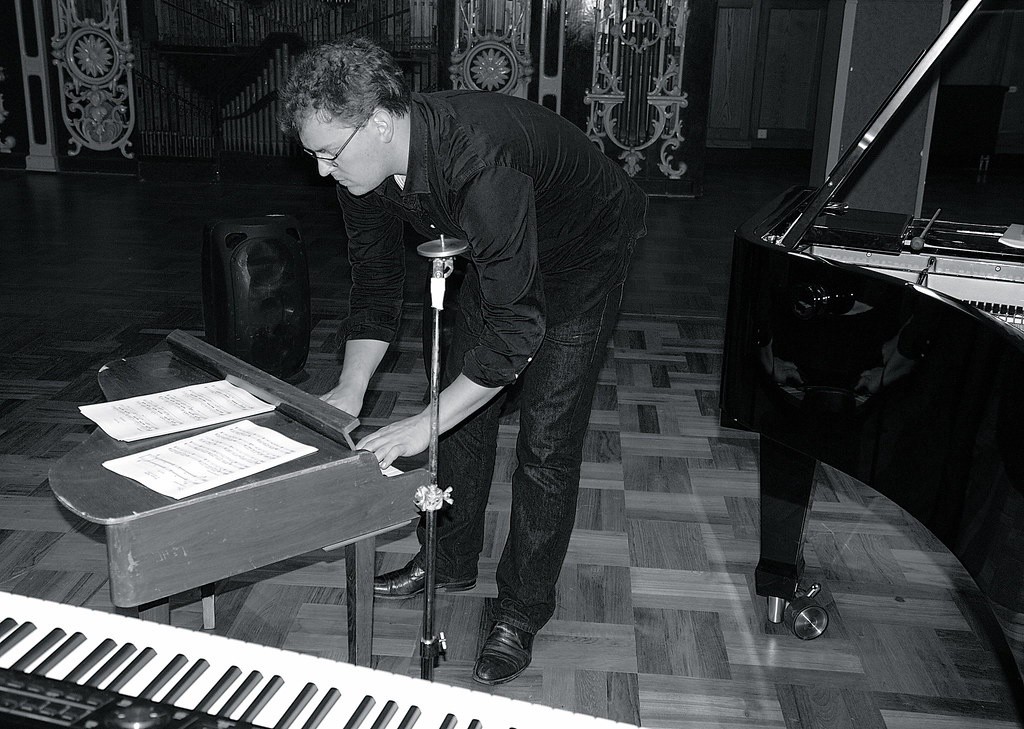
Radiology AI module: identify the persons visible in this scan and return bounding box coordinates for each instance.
[275,35,648,685]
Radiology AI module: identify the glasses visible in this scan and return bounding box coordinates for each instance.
[299,118,364,168]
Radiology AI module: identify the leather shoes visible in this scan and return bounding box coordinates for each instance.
[473,621,535,686]
[373,559,477,599]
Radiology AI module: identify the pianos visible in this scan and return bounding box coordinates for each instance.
[0,589,654,729]
[46,329,431,671]
[718,0,1024,729]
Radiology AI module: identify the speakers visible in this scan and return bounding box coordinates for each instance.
[201,213,311,381]
